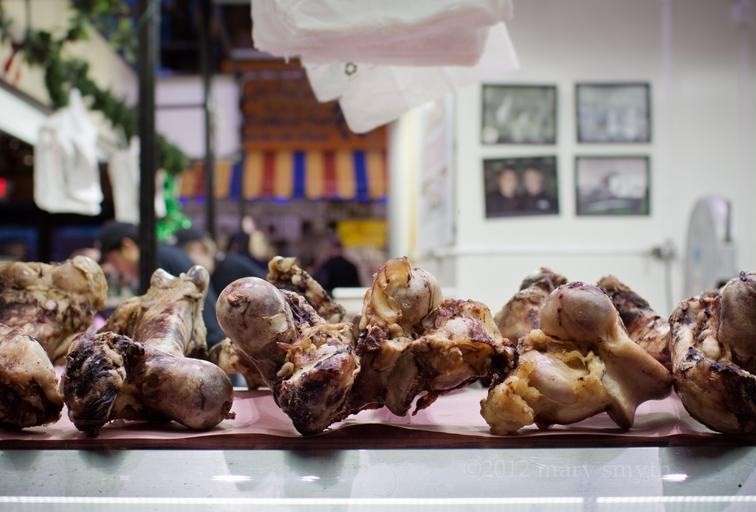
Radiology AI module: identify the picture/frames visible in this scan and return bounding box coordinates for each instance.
[480,81,654,217]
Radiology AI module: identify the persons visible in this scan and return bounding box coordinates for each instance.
[485,163,525,215]
[521,166,559,213]
